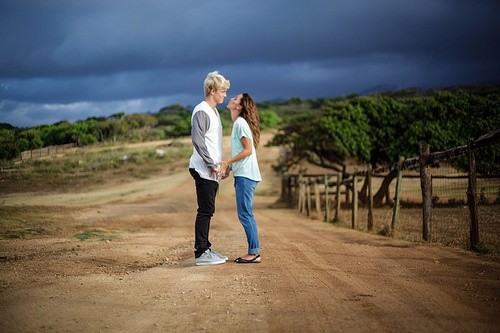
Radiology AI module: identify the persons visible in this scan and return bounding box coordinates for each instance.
[188,71,230,266]
[219,93,263,263]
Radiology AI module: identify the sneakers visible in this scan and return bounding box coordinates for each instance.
[195,249,228,265]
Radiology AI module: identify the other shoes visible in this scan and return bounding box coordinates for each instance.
[234,253,262,263]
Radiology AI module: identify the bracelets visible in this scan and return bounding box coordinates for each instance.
[207,165,217,168]
